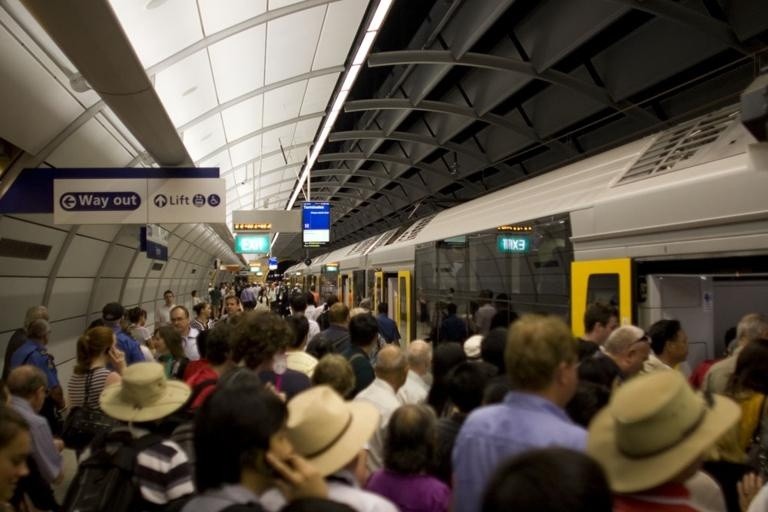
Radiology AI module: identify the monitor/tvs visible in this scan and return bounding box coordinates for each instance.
[235,234,270,254]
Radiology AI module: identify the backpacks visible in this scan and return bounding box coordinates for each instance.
[60,426,163,512]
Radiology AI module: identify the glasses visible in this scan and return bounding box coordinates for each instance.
[628,331,648,347]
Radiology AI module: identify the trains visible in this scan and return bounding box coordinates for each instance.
[282,74,767,373]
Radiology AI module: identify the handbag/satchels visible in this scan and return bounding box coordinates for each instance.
[60,366,106,450]
[747,394,767,477]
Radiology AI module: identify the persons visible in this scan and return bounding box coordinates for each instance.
[1,279,767,510]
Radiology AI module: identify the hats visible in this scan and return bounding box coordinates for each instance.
[98,361,192,423]
[462,334,484,364]
[282,384,380,478]
[585,368,742,495]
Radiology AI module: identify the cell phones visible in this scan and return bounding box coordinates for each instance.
[107,346,116,355]
